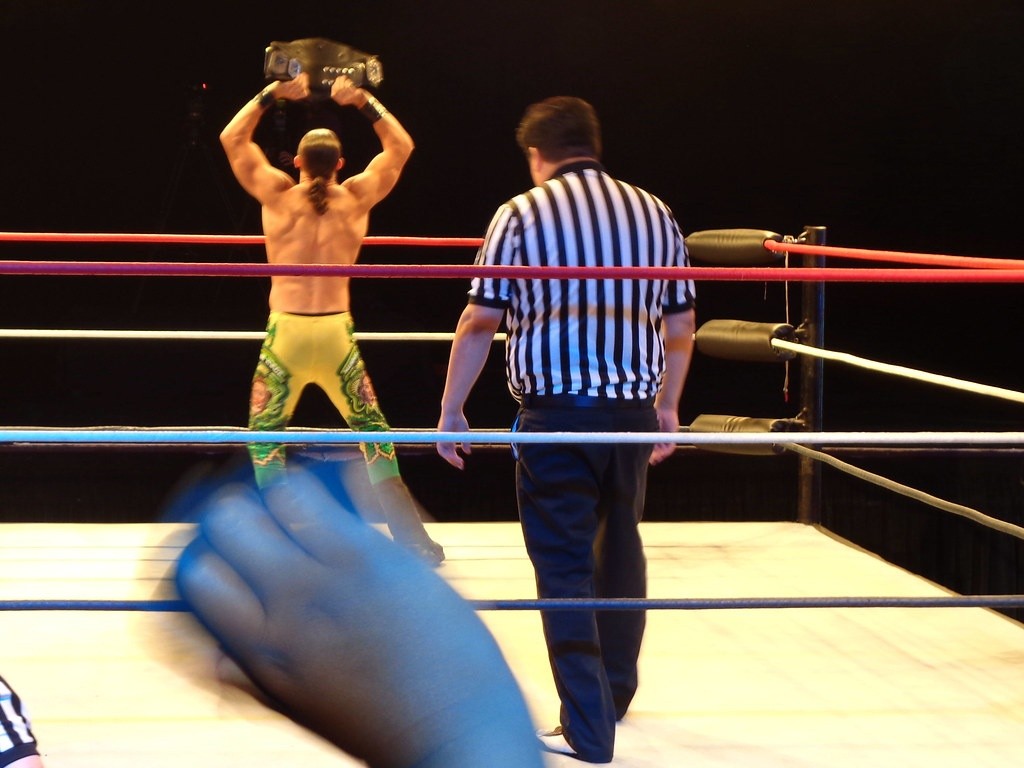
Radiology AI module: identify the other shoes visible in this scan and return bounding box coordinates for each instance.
[535,728,584,760]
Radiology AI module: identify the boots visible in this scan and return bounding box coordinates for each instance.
[373,475,445,563]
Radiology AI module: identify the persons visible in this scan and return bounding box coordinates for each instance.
[172,460,546,768]
[0,675,45,768]
[220,73,446,566]
[438,96,696,763]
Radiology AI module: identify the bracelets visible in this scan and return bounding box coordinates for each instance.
[255,91,277,109]
[360,97,388,123]
